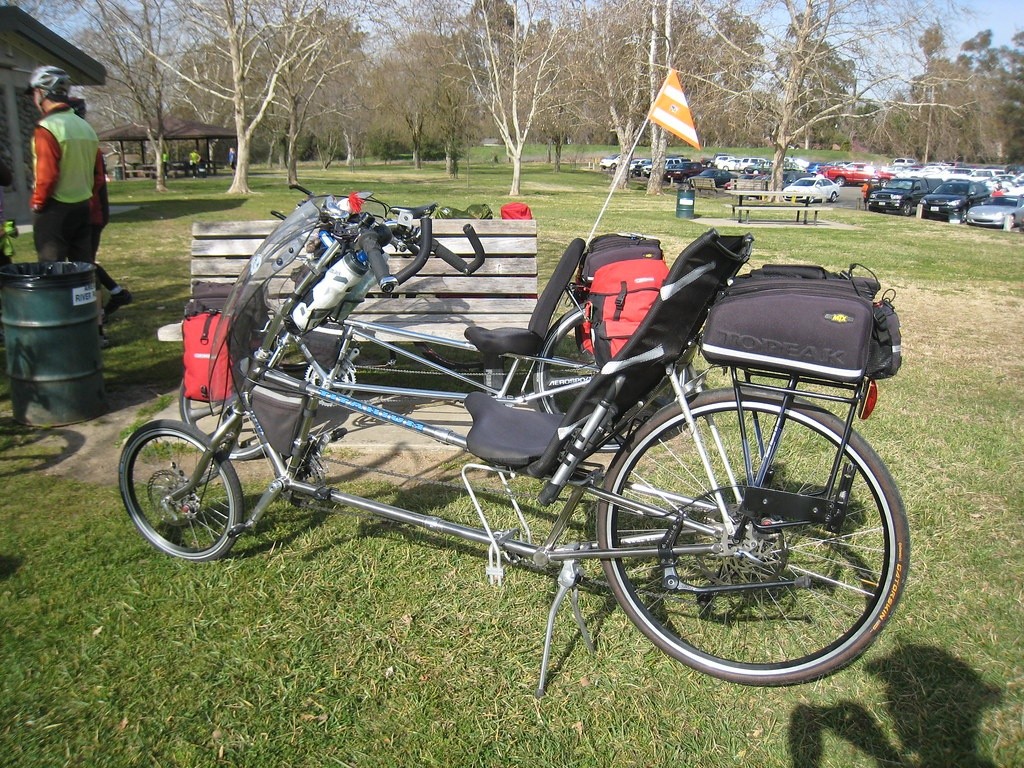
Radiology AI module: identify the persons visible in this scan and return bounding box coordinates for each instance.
[188,149,201,178]
[24,65,100,263]
[862,181,869,210]
[992,188,1002,196]
[229,146,237,169]
[69,97,133,313]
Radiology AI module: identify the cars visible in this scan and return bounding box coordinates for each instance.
[599,151,1024,231]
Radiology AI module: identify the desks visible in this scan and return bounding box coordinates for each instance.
[730,192,816,224]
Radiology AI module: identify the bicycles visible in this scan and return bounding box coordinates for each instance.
[118,185,913,699]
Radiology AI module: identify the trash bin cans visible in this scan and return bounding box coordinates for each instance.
[0,261,106,428]
[675,189,695,219]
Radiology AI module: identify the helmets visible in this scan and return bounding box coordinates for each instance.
[24,66,70,97]
[70,97,85,119]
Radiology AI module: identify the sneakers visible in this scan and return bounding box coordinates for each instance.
[101,308,108,323]
[105,289,132,315]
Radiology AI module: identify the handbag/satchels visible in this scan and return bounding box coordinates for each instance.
[699,262,901,382]
[307,319,345,368]
[180,279,268,403]
[570,233,671,370]
[248,381,310,457]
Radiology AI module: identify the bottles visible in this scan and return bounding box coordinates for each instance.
[290,250,368,331]
[329,252,391,320]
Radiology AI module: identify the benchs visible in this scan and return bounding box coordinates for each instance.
[692,178,724,195]
[158,220,538,393]
[725,204,833,226]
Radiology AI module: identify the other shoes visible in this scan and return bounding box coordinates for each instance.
[99,333,110,349]
